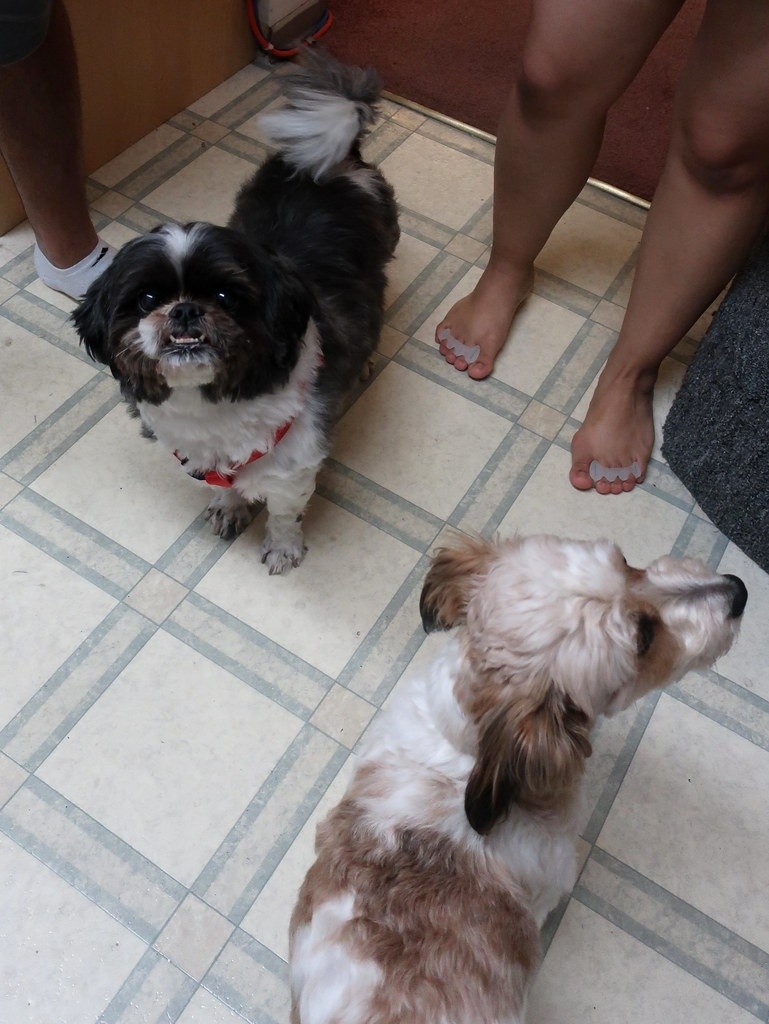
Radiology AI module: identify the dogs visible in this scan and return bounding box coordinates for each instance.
[72,38,401,576]
[287,536,748,1024]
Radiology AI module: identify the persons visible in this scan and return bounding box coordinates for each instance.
[434,0,769,494]
[0,0,119,304]
